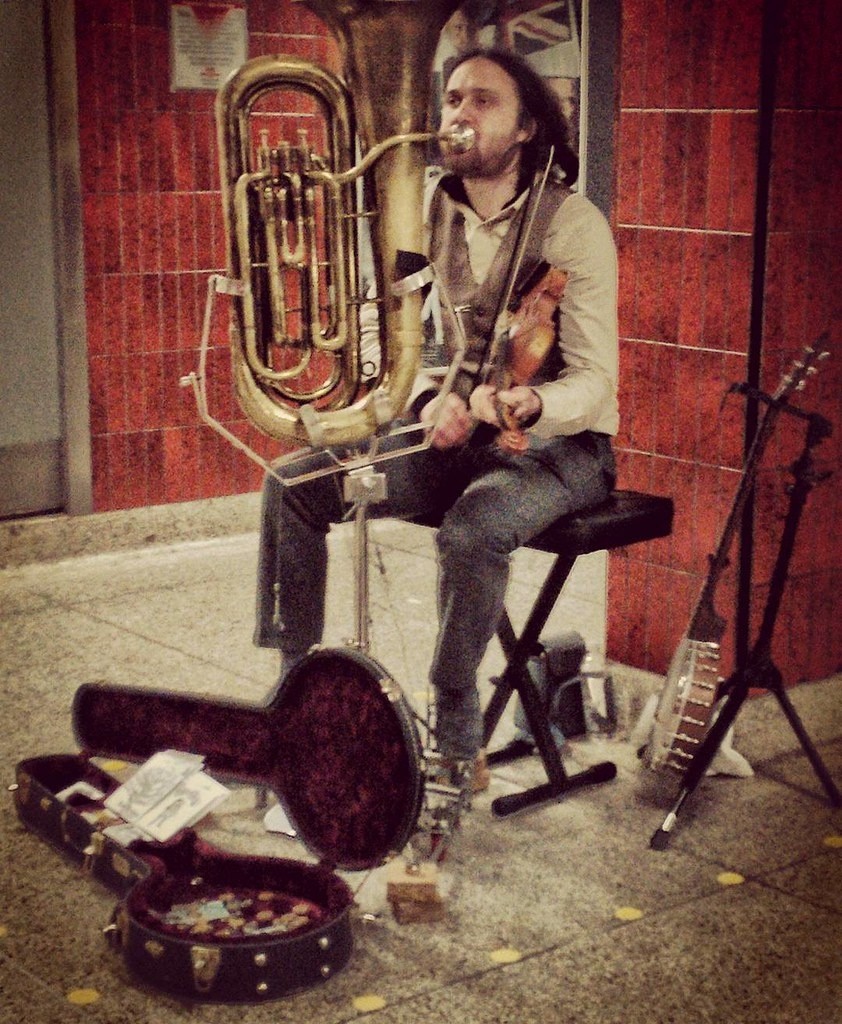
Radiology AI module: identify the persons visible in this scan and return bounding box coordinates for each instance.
[245,48,618,801]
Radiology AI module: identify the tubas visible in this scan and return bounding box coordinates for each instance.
[211,0,479,455]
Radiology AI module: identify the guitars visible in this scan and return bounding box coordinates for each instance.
[640,330,833,779]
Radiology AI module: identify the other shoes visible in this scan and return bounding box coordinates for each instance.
[425,753,491,794]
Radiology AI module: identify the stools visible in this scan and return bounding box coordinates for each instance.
[397,489,677,813]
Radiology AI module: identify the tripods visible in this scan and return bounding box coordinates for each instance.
[646,382,841,849]
